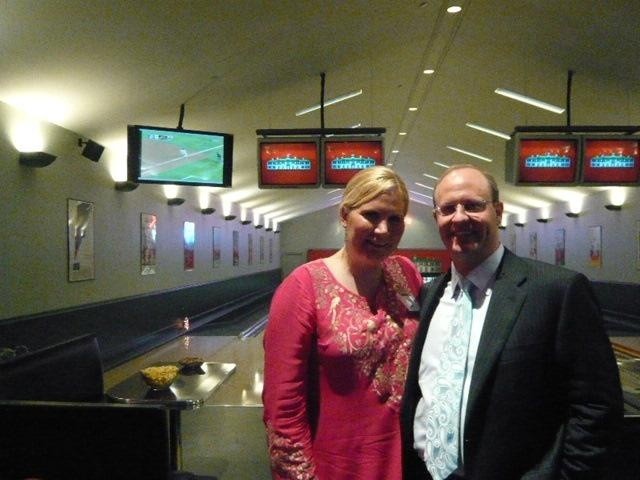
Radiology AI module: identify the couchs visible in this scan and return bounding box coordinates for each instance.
[0,333,169,480]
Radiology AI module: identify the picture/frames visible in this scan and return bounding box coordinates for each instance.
[140,211,157,275]
[183,222,273,272]
[66,198,95,281]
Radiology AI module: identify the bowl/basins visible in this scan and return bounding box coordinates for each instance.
[140,365,180,392]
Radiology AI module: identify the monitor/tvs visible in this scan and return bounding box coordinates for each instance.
[583,133,640,188]
[126,125,234,193]
[323,134,385,189]
[505,133,581,187]
[257,135,319,190]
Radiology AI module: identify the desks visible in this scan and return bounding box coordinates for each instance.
[107,360,236,480]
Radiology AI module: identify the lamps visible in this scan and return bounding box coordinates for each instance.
[115,181,138,192]
[78,137,105,162]
[499,205,621,230]
[18,152,56,167]
[202,207,280,234]
[168,198,185,206]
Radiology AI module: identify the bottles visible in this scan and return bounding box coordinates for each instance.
[413,256,442,275]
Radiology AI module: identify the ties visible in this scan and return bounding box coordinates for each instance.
[423,279,473,480]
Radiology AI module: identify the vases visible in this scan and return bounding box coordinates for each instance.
[509,226,602,267]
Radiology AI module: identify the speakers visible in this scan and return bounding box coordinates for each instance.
[82,139,106,162]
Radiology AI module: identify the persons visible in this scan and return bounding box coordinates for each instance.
[262,166,425,480]
[180,148,188,162]
[399,163,625,480]
[216,151,221,161]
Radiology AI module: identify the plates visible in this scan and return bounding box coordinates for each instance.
[179,357,205,368]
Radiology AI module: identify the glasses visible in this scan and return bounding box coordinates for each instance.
[433,199,492,216]
